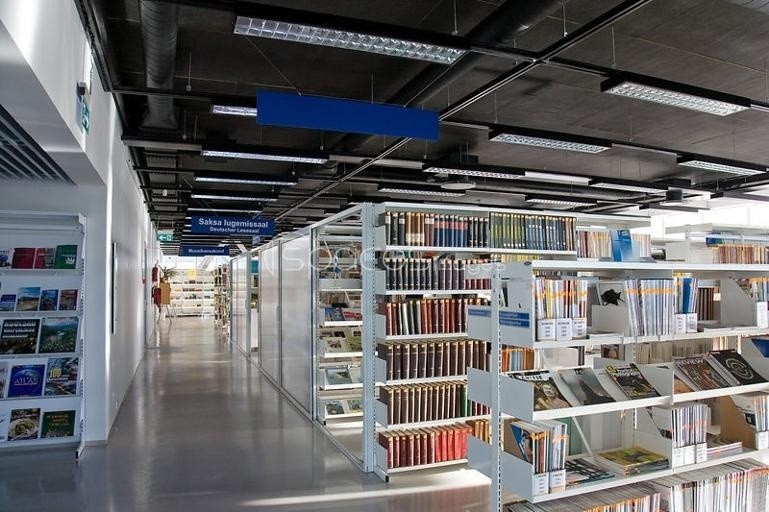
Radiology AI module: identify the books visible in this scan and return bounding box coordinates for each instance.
[171,266,232,331]
[372,206,769,512]
[310,211,372,424]
[249,259,260,311]
[1,244,79,443]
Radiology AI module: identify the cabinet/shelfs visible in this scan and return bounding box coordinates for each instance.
[0,208,87,459]
[170,274,216,317]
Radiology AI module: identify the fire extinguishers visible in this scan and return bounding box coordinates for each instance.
[152,264,158,281]
[151,283,161,305]
